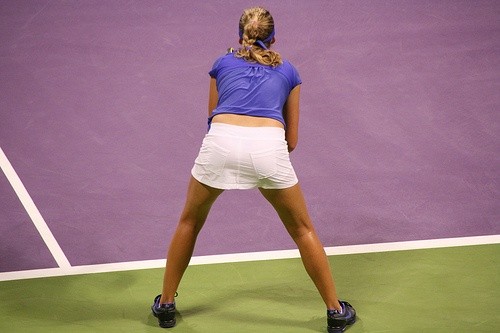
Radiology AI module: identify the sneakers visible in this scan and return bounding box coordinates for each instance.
[152,294,178,327]
[327,300,356,333]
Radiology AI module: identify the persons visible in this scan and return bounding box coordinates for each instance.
[152,7,358,332]
